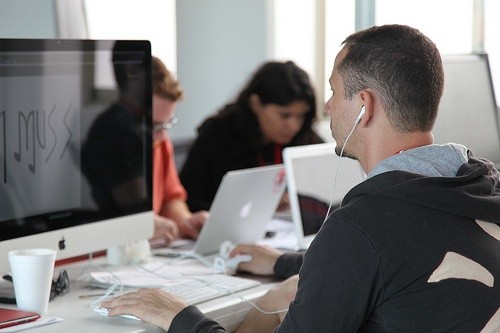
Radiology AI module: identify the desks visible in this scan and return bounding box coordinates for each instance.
[0,206,299,333]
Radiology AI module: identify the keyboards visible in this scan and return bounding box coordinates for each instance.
[93,273,261,320]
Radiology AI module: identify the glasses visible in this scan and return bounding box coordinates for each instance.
[153,117,178,133]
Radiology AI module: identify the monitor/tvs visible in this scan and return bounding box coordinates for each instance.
[0,38,155,279]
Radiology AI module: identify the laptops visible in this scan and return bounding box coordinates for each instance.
[284,141,366,252]
[154,165,287,258]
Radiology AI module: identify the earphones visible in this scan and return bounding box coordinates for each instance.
[354,106,365,125]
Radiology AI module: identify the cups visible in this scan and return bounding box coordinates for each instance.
[9,249,57,314]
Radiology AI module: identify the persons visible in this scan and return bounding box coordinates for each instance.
[179,61,327,213]
[100,25,500,333]
[81,41,147,210]
[152,56,210,245]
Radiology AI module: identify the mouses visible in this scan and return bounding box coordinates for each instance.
[225,256,254,275]
